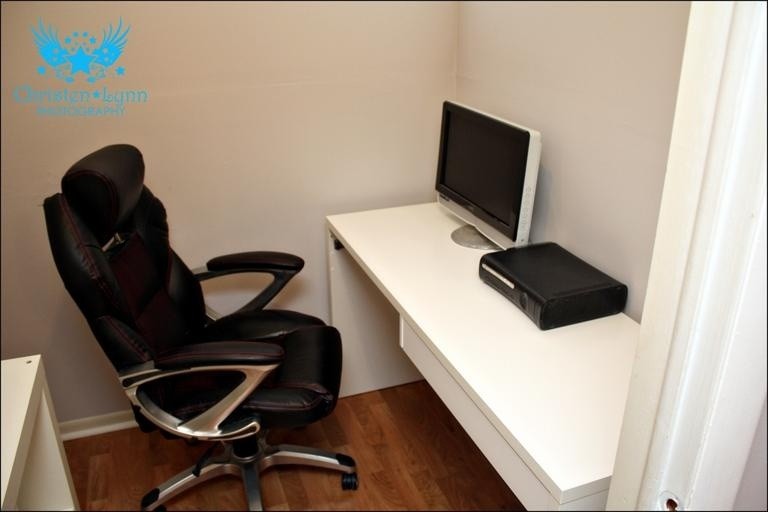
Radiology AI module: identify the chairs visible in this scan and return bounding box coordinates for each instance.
[43,142,361,512]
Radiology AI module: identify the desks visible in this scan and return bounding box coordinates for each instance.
[327,204,640,511]
[1,355,79,510]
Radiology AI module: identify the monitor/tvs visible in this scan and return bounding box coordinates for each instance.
[433,101,543,250]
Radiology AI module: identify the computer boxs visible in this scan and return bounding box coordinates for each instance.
[479,241,628,329]
